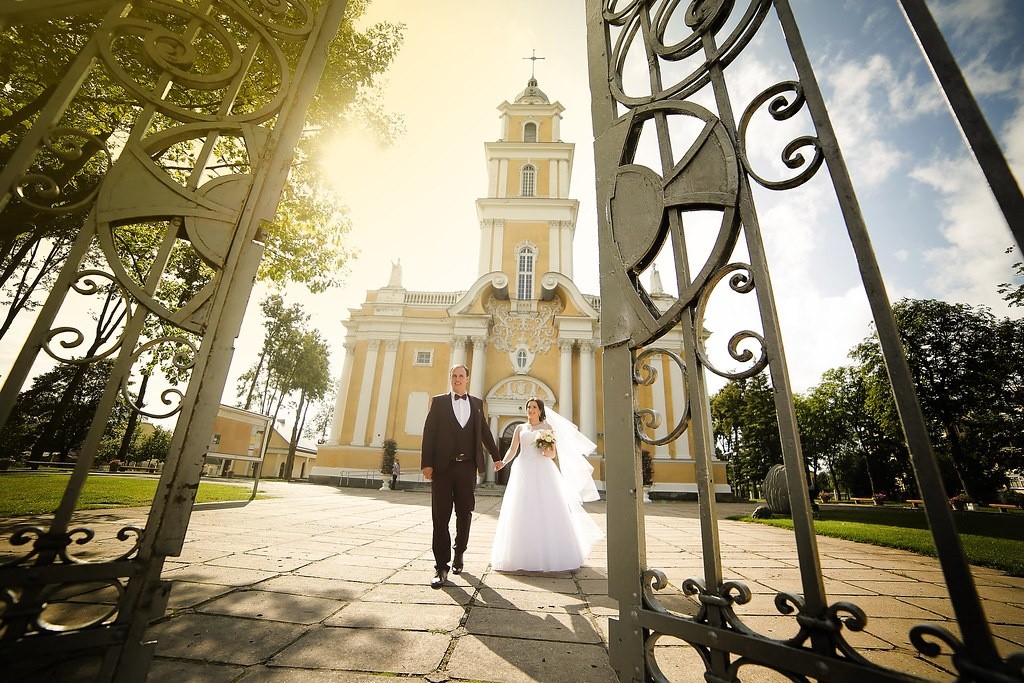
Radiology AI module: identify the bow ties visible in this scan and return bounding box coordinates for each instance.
[455,394,466,401]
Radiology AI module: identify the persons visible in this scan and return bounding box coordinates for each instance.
[391,459,400,490]
[494,397,556,574]
[421,364,503,589]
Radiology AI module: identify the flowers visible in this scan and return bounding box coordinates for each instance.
[872,492,885,499]
[949,496,964,505]
[534,430,555,453]
[818,491,830,498]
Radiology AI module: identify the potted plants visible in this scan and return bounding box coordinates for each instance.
[641,449,654,504]
[379,438,396,491]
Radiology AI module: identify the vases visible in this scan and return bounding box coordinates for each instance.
[821,499,829,503]
[873,499,884,506]
[951,504,964,511]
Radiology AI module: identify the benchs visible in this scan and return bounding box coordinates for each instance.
[989,503,1017,513]
[29,460,77,468]
[850,497,873,504]
[903,499,924,510]
[120,466,158,473]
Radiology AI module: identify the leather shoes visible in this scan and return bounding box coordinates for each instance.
[452,551,464,574]
[430,565,448,588]
